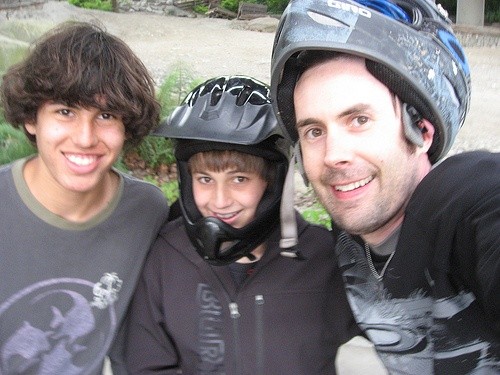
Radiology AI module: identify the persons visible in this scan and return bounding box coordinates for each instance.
[269,0,500,375]
[0,23,170,375]
[107,73,371,375]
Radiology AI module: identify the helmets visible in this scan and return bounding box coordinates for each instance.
[267,1,474,166]
[147,73,296,268]
